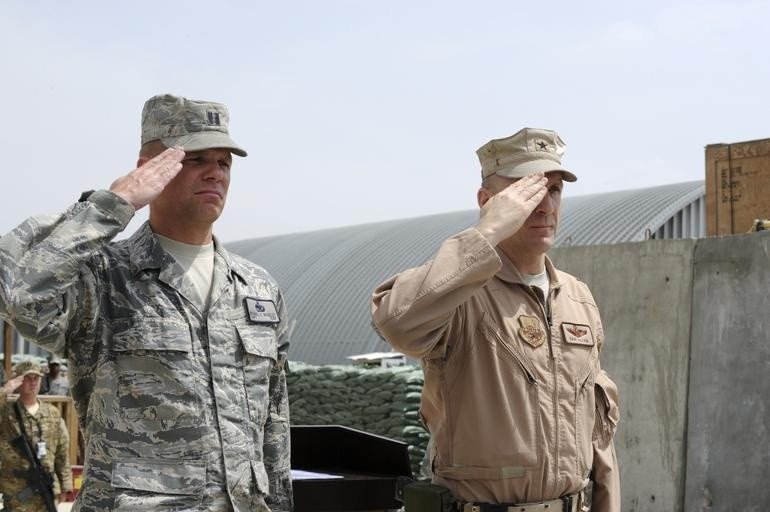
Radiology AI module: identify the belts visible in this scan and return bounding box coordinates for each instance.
[451,491,590,512]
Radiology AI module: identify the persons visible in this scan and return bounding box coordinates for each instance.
[40,356,69,398]
[370,127,626,511]
[1,361,74,512]
[0,93,296,512]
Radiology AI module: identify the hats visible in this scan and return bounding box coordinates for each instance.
[476,126,578,183]
[0,352,68,377]
[141,92,248,158]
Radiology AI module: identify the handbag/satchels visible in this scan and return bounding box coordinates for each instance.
[402,481,450,512]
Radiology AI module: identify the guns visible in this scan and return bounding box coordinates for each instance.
[8,402,55,512]
[394,476,433,502]
